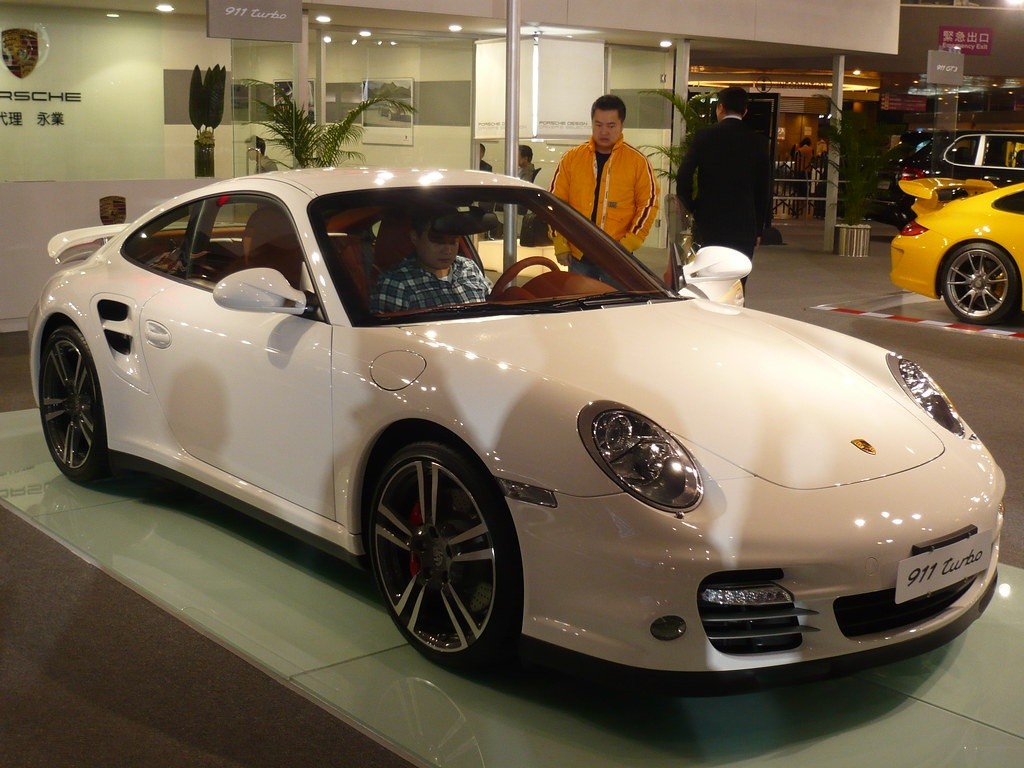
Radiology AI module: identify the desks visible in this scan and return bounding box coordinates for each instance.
[775,178,852,218]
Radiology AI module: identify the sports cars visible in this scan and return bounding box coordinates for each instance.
[27,164,1006,678]
[889,178,1023,326]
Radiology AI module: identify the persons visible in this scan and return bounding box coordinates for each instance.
[676,87,771,297]
[547,95,660,284]
[244,135,279,174]
[368,203,492,315]
[796,137,814,174]
[517,145,535,181]
[242,205,333,287]
[480,144,492,171]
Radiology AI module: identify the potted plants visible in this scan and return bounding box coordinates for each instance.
[188,64,227,178]
[634,87,721,263]
[810,94,917,259]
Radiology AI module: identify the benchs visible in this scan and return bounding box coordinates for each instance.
[771,196,848,217]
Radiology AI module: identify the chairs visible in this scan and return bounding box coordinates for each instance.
[241,204,473,317]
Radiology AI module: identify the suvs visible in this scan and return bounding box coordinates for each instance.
[867,129,1024,231]
